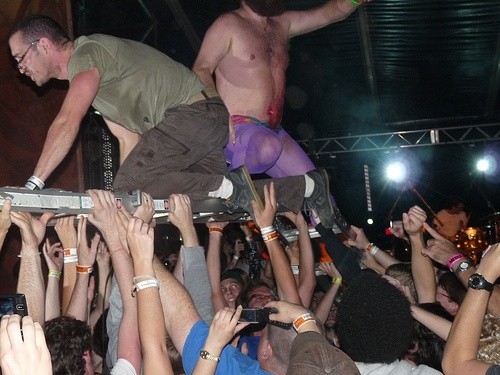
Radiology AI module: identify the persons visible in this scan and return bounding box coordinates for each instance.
[0,183,500,375]
[7,16,335,231]
[190,0,363,224]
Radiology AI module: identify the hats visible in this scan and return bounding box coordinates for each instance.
[286,331,361,375]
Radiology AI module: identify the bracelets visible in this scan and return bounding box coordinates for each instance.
[260,226,278,242]
[47,269,62,279]
[330,277,342,285]
[76,265,93,274]
[455,261,473,274]
[25,176,45,190]
[131,279,161,292]
[232,255,238,260]
[351,0,362,7]
[246,235,253,241]
[134,275,154,284]
[366,243,379,255]
[292,313,316,331]
[17,252,41,258]
[199,349,221,362]
[63,248,78,257]
[290,265,300,275]
[64,256,78,264]
[209,227,223,236]
[447,254,466,273]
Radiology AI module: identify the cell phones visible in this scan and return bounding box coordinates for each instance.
[237,307,293,330]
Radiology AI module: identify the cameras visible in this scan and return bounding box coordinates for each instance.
[0,293,28,342]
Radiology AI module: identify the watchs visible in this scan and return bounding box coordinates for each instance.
[468,273,494,292]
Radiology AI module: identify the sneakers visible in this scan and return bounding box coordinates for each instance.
[222,164,264,229]
[303,169,334,229]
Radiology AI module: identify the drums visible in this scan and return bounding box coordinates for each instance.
[457,225,491,253]
[487,211,500,244]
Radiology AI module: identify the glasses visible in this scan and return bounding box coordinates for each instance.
[15,40,39,70]
